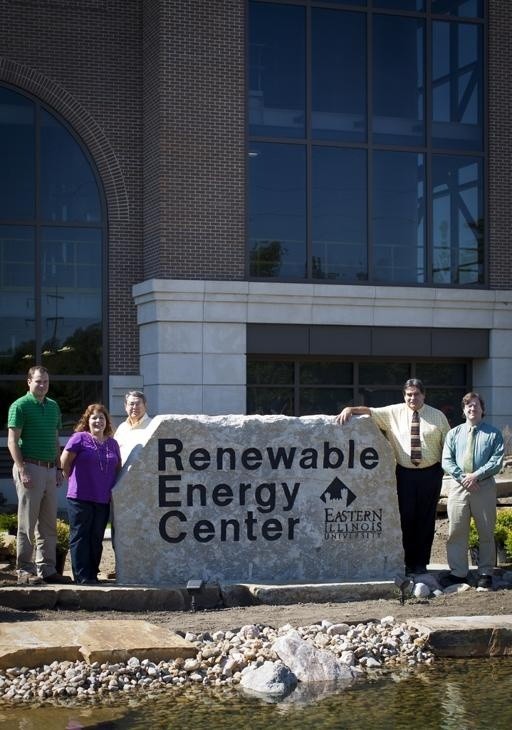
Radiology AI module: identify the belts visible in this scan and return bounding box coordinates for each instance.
[24,459,55,468]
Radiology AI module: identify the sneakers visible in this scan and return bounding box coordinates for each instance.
[406,566,427,574]
[18,573,99,585]
[478,575,492,587]
[440,574,468,586]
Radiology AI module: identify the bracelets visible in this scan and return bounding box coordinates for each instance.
[57,467,64,471]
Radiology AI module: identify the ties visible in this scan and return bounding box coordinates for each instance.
[411,410,423,466]
[465,426,474,478]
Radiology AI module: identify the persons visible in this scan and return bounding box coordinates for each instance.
[440,392,505,589]
[107,390,153,577]
[334,378,452,575]
[58,403,122,585]
[7,366,73,585]
